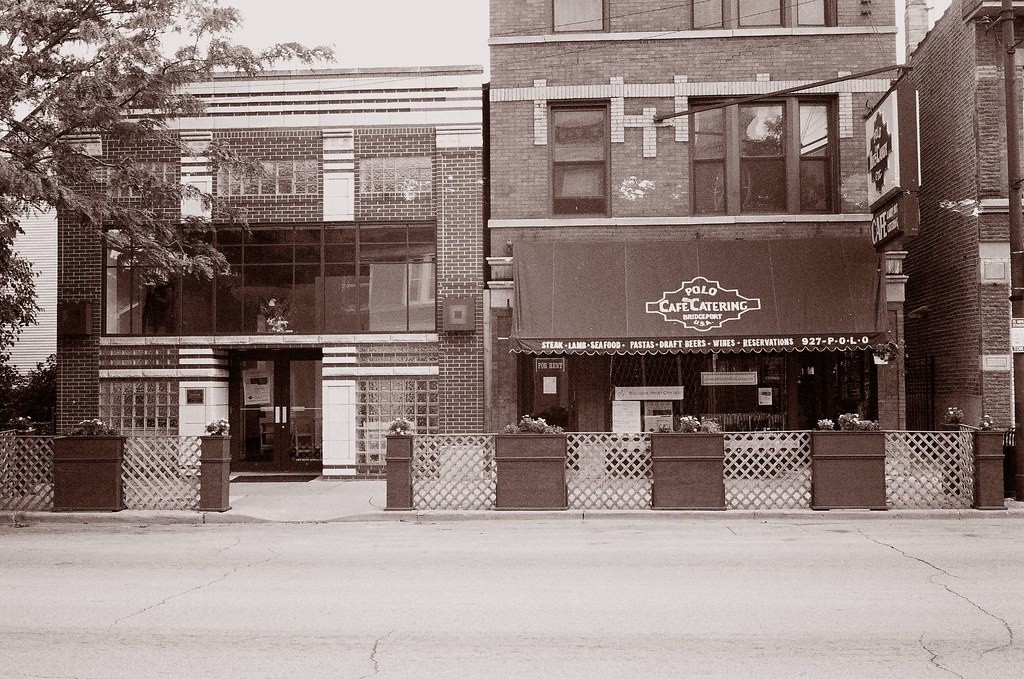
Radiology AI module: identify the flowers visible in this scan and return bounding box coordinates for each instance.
[943,407,964,424]
[649,415,719,433]
[874,330,909,362]
[205,418,230,436]
[819,414,881,430]
[979,416,996,430]
[499,415,563,434]
[388,418,413,435]
[68,418,120,436]
[7,415,35,430]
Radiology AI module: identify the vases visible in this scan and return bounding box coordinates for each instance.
[873,354,889,365]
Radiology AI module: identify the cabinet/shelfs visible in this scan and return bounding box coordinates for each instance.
[291,415,313,457]
[259,416,275,453]
